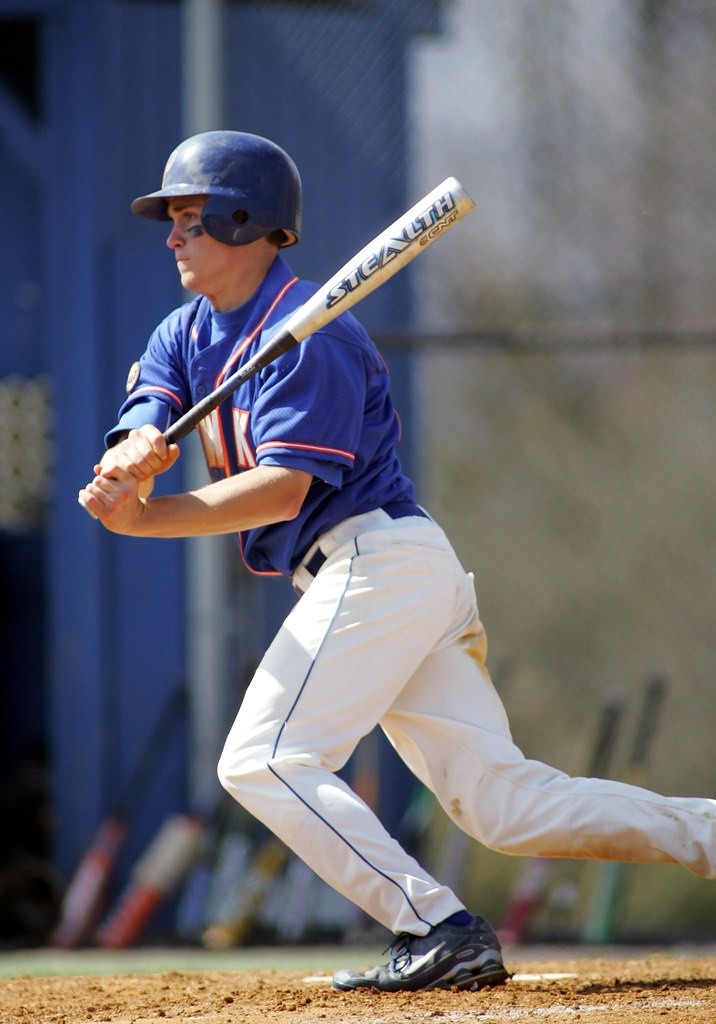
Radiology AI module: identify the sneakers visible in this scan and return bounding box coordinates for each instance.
[332,915,510,993]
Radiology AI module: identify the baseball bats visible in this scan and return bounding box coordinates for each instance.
[75,174,477,523]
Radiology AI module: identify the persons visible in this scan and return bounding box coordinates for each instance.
[81,132,716,996]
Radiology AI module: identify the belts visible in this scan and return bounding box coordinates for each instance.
[293,547,327,598]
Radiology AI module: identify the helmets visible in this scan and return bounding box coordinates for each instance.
[131,130,302,249]
